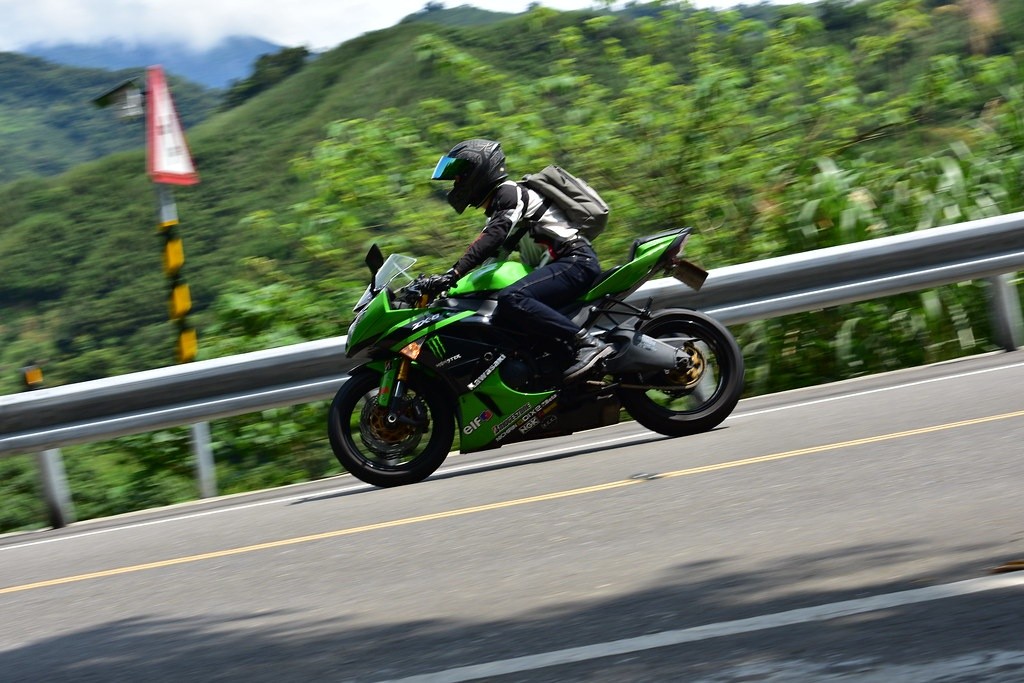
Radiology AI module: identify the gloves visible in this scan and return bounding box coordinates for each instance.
[416,269,458,298]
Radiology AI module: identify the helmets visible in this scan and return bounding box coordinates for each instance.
[445,138,510,216]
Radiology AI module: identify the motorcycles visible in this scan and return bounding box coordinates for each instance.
[327,224,745,487]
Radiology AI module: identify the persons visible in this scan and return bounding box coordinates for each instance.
[404,137,616,381]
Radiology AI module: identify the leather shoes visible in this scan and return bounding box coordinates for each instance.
[561,327,614,380]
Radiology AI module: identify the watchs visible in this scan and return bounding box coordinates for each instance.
[447,268,460,281]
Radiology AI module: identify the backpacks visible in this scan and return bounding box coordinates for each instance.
[515,164,610,243]
